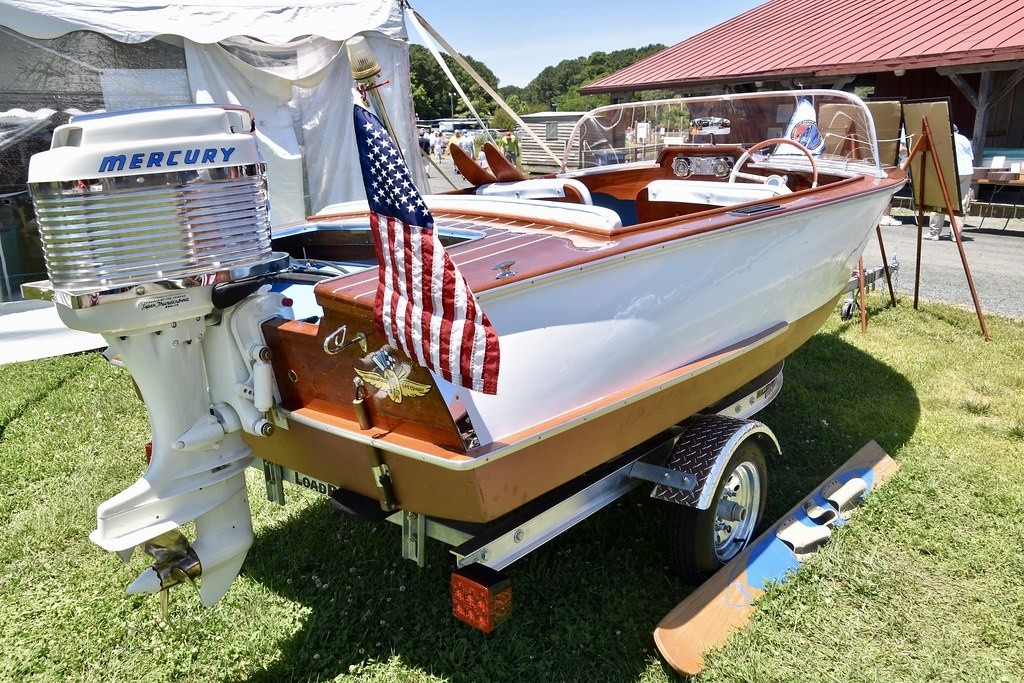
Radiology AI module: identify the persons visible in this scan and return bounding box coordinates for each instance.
[898,128,910,176]
[475,145,489,171]
[881,202,903,227]
[420,127,444,164]
[418,132,431,178]
[921,124,974,241]
[501,132,520,164]
[447,129,475,174]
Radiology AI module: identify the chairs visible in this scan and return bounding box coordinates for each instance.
[635,180,788,226]
[475,178,594,206]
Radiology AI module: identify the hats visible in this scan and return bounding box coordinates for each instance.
[506,131,511,139]
[461,129,466,135]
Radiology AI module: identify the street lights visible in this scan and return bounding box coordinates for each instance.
[448,91,454,123]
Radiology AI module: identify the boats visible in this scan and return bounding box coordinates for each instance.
[19,87,913,610]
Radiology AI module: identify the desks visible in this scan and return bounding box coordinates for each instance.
[969,172,1024,234]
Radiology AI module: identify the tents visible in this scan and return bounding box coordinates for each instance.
[0,0,432,226]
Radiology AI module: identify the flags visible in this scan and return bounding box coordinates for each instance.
[772,98,826,155]
[352,87,500,396]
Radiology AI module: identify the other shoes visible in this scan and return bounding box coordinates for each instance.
[922,233,939,241]
[425,173,430,179]
[880,215,902,226]
[951,236,956,242]
